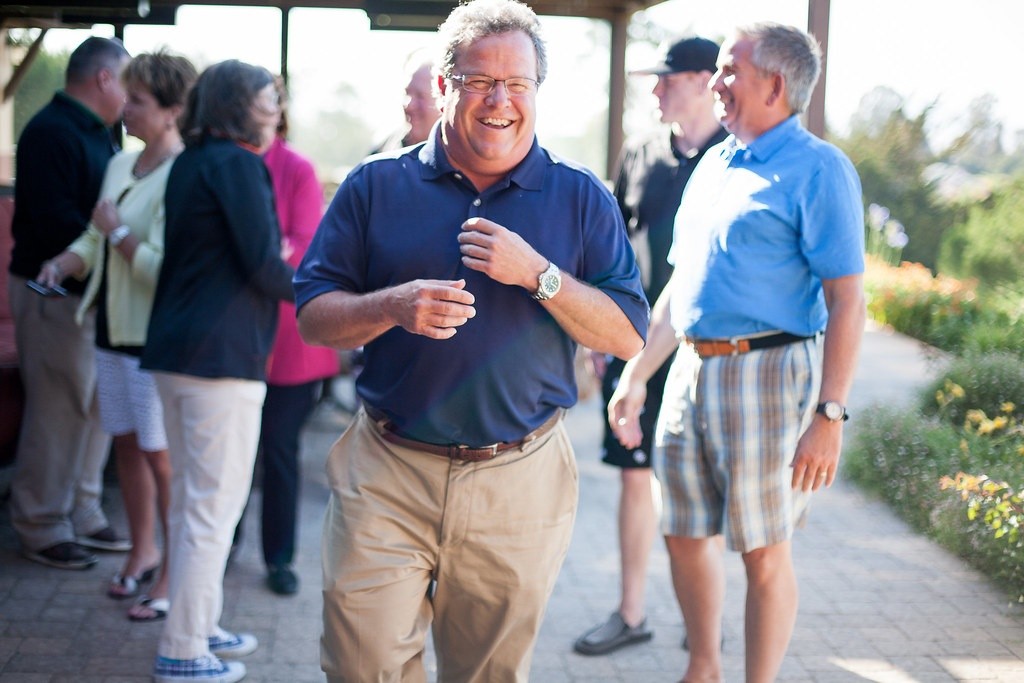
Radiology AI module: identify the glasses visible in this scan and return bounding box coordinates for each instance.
[443,72,540,97]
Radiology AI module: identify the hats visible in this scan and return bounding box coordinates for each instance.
[648,37,720,76]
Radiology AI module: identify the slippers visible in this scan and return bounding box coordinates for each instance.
[109,565,157,599]
[127,598,169,620]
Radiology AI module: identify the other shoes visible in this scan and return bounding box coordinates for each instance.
[268,564,297,595]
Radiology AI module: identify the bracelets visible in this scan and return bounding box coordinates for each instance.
[110,225,132,247]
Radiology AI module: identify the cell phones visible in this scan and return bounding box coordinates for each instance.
[27,280,69,298]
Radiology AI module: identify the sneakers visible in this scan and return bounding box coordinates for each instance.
[683,635,725,650]
[153,651,246,683]
[575,613,651,655]
[74,528,134,551]
[208,633,257,657]
[23,542,99,569]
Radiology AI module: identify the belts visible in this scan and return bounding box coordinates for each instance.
[685,330,823,357]
[363,402,561,461]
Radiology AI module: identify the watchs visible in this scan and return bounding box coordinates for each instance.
[816,400,849,424]
[532,260,562,301]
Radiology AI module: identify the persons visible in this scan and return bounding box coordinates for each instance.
[8,0,864,683]
